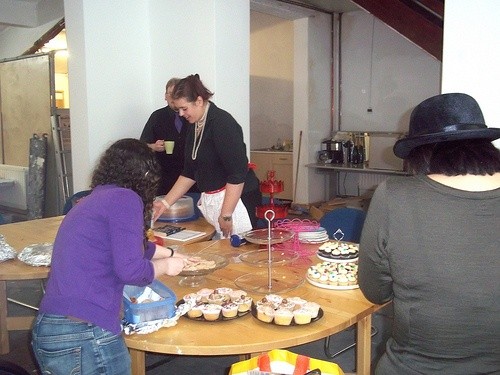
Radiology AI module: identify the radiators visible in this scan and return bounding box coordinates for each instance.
[0,164,28,211]
[358,173,390,191]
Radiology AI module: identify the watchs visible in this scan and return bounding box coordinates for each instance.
[220,214,232,221]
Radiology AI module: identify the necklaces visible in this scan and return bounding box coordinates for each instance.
[192,113,205,160]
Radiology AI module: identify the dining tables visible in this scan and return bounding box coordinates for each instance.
[121,239,392,375]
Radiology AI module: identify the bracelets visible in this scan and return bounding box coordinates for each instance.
[161,199,170,209]
[167,247,174,257]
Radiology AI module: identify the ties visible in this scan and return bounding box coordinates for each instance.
[174,113,183,134]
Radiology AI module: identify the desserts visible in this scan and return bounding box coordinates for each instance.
[317,242,359,259]
[307,261,359,287]
[256,294,320,326]
[183,287,253,321]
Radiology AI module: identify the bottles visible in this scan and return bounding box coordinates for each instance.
[319,131,370,165]
[230,232,248,247]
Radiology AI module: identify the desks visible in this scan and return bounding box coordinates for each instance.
[305,163,410,201]
[0,215,65,354]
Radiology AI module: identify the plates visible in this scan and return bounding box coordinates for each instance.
[252,304,324,327]
[177,297,254,322]
[296,224,329,244]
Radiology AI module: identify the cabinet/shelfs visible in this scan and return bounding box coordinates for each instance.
[252,153,293,200]
[366,134,408,171]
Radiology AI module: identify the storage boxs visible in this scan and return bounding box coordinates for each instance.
[123,279,177,324]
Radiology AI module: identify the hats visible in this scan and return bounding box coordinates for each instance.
[393,93,500,159]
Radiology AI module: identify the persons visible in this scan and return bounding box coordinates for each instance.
[32,138,201,375]
[139,78,201,196]
[153,74,261,241]
[357,92,500,375]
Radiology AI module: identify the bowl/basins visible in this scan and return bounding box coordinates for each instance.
[123,281,176,324]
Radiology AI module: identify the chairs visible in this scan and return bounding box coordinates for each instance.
[256,197,280,228]
[319,208,366,244]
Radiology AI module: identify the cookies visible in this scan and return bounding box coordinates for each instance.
[182,257,216,270]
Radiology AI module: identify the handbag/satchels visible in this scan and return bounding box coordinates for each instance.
[229,349,345,375]
